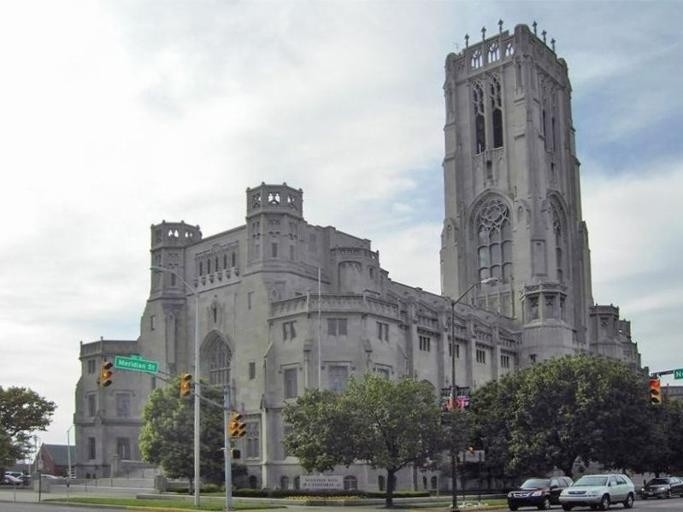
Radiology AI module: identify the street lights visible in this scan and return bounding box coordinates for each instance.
[147,264,204,506]
[449,276,501,510]
[65,423,79,479]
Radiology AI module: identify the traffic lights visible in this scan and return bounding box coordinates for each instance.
[230,447,241,460]
[228,421,239,438]
[649,378,661,405]
[231,413,242,421]
[179,370,192,397]
[238,422,247,437]
[99,360,113,387]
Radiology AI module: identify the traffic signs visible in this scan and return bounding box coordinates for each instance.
[113,355,159,375]
[673,368,683,379]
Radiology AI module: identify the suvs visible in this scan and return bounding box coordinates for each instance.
[558,473,636,511]
[506,474,575,511]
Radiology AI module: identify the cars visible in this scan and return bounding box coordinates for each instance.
[639,475,682,501]
[0,468,65,486]
